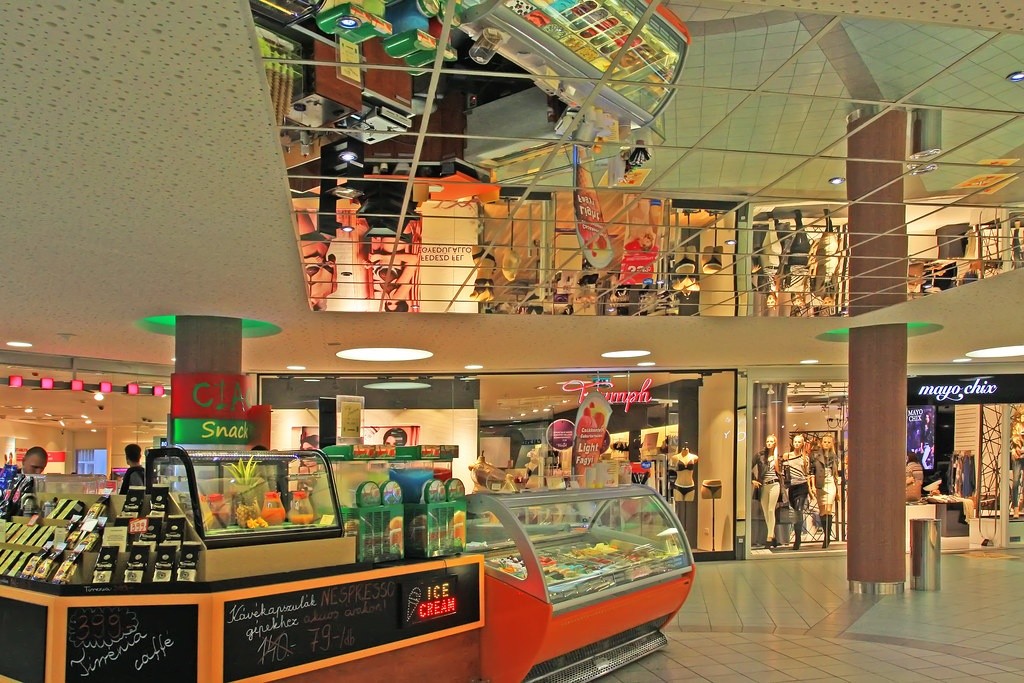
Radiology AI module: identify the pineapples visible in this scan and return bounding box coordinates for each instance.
[223,456,260,527]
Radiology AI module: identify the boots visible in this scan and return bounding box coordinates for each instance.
[791,523,801,550]
[820,514,832,547]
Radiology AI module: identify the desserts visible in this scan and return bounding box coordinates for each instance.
[492,542,649,584]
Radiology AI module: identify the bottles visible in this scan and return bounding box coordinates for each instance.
[210,493,227,530]
[617,460,632,485]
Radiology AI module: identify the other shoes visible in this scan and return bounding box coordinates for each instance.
[1019,507,1024,515]
[1013,507,1019,519]
[765,541,771,549]
[772,538,777,547]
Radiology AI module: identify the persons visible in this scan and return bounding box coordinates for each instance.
[752,434,781,549]
[783,434,814,550]
[906,452,923,501]
[701,480,721,499]
[119,444,144,494]
[16,446,48,474]
[296,209,336,312]
[672,447,698,501]
[470,246,497,303]
[703,246,723,274]
[251,445,278,490]
[525,452,541,488]
[360,237,418,313]
[811,434,840,548]
[672,245,700,301]
[752,209,842,315]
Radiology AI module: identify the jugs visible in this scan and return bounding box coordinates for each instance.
[200,495,212,528]
[289,491,312,524]
[261,492,285,525]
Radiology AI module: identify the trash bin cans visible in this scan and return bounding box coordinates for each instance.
[909,518,942,592]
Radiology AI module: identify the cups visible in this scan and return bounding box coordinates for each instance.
[592,109,603,131]
[471,462,506,491]
[597,113,611,137]
[586,469,595,489]
[594,463,606,488]
[468,27,504,64]
[586,106,597,124]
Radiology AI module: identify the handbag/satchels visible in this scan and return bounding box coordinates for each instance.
[905,466,915,485]
[783,465,791,484]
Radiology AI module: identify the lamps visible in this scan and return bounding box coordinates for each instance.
[766,383,775,394]
[6,373,164,397]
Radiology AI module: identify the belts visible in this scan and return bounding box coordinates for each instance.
[766,478,779,484]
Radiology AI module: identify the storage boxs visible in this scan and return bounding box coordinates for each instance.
[341,503,405,564]
[407,496,465,558]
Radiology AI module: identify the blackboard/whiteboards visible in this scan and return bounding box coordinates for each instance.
[212,554,485,683]
[0,585,54,683]
[52,593,213,683]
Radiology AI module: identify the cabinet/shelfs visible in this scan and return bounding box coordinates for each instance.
[458,0,690,132]
